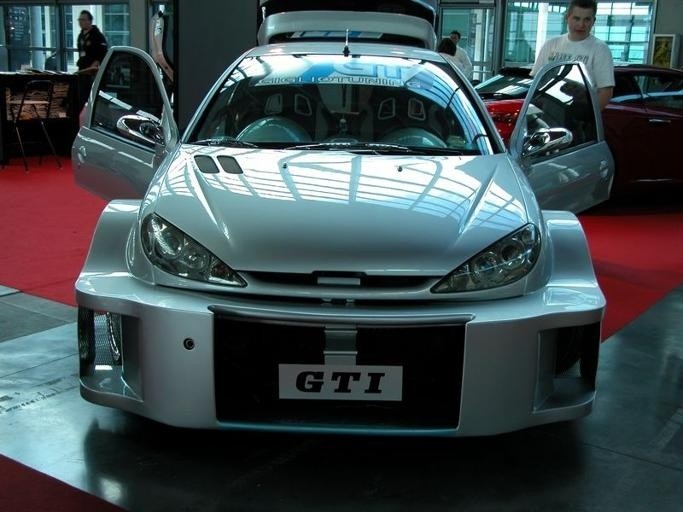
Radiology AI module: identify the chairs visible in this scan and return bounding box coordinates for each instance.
[5,79,64,174]
[243,84,450,143]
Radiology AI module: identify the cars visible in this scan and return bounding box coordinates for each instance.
[474,62,683,189]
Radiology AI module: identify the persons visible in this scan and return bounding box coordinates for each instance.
[435,30,473,80]
[529,0,615,142]
[76,10,107,71]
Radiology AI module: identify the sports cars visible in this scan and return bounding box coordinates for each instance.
[70,1,615,437]
